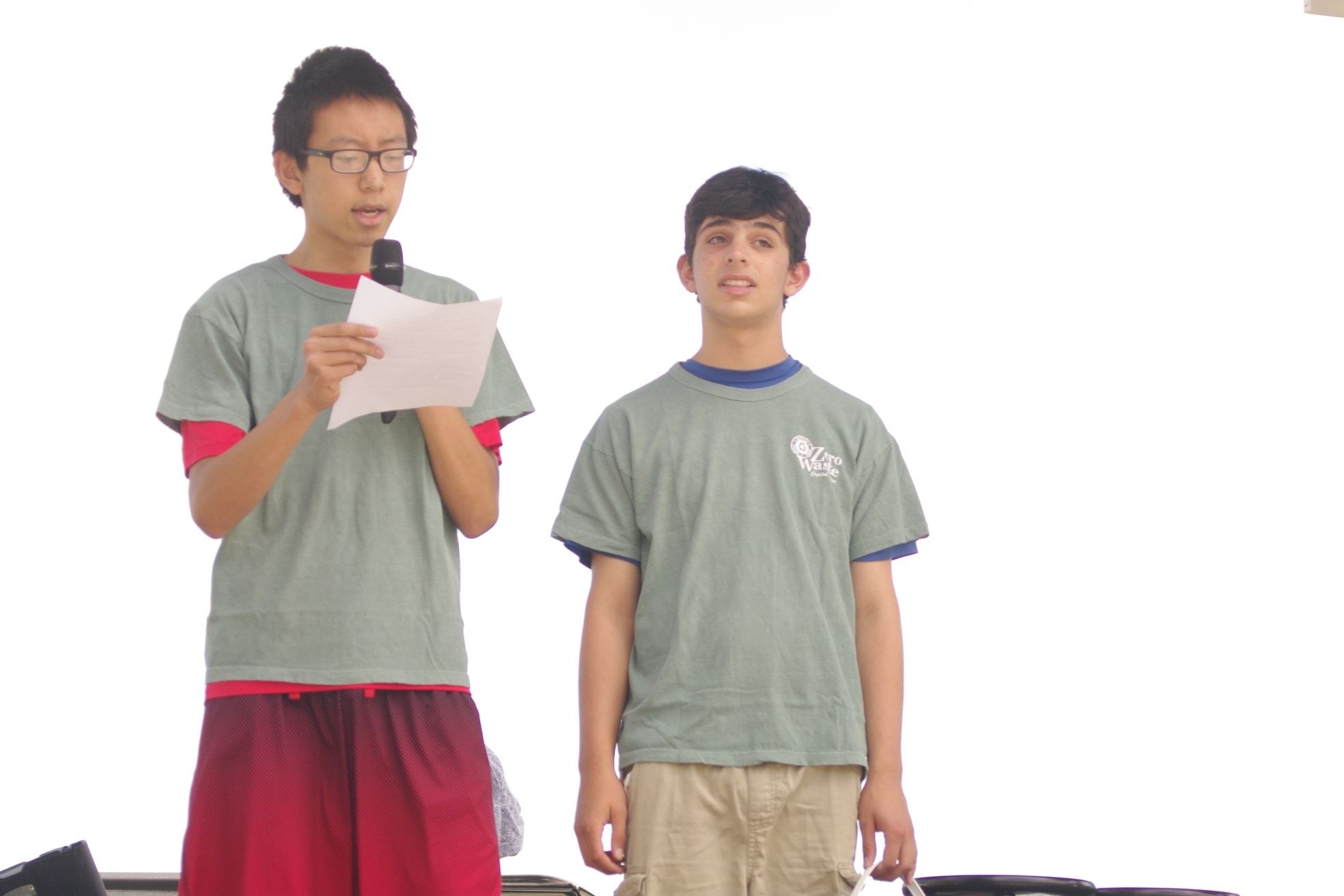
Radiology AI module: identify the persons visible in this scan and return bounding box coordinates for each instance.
[182,47,501,896]
[572,163,918,896]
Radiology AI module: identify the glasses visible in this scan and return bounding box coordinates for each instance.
[297,145,417,174]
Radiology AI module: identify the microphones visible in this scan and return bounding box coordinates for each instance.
[368,239,404,423]
